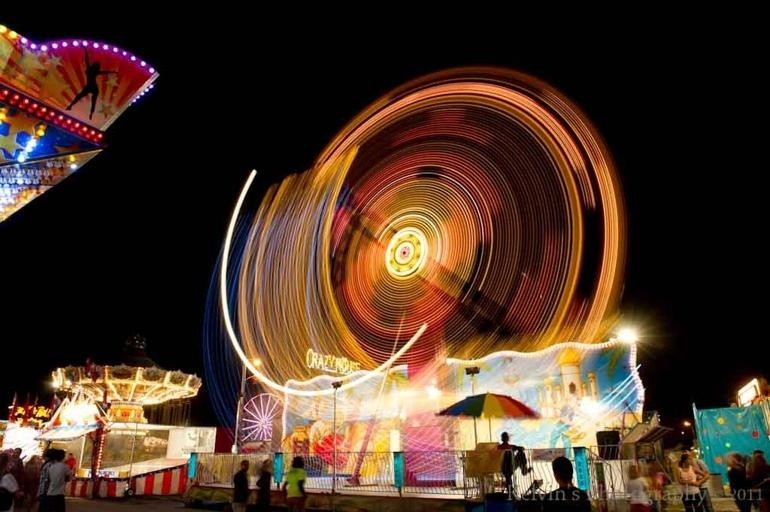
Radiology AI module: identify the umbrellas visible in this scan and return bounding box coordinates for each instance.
[434,391,543,442]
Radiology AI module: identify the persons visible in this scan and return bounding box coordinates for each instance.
[724,451,754,511]
[547,397,578,464]
[676,453,710,511]
[742,453,760,509]
[540,456,594,510]
[625,464,653,512]
[230,459,250,512]
[745,449,770,512]
[680,445,716,511]
[496,431,533,492]
[60,452,77,494]
[0,445,71,512]
[280,454,308,511]
[254,459,273,511]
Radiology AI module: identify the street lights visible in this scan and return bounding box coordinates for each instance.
[331,380,344,492]
[682,419,697,446]
[231,358,263,485]
[465,364,481,449]
